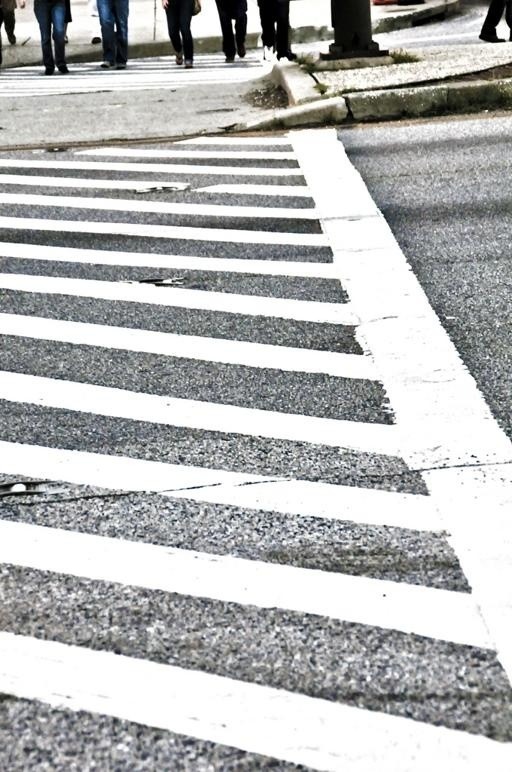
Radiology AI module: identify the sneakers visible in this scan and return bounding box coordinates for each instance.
[176,56,184,65]
[117,63,126,69]
[64,36,68,43]
[185,59,193,68]
[101,60,111,68]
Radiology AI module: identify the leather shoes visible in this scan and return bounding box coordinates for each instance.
[478,32,505,43]
[45,65,55,75]
[8,34,16,45]
[226,48,236,63]
[58,66,69,73]
[237,46,245,58]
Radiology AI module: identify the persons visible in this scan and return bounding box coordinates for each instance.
[257,0,297,64]
[0,0,27,64]
[214,0,249,63]
[160,0,202,68]
[34,0,72,75]
[479,0,512,43]
[96,0,129,69]
